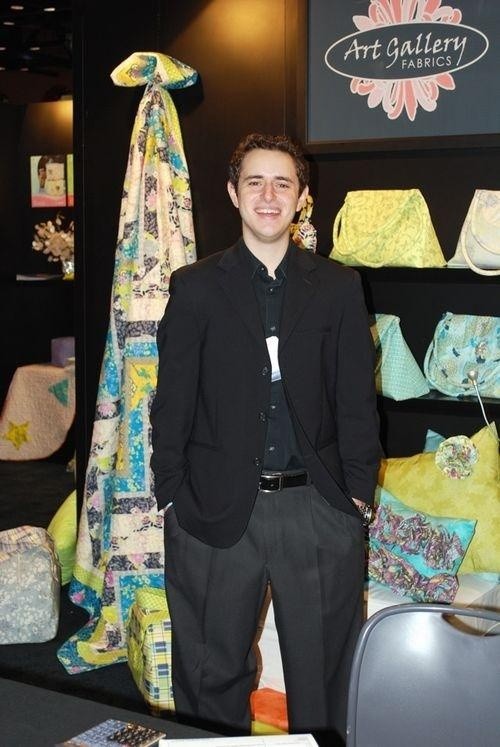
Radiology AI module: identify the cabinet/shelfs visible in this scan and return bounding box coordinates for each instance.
[345,260,500,417]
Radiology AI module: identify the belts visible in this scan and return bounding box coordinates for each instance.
[258,472,307,495]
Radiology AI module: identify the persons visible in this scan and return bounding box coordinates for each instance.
[149,131,382,747]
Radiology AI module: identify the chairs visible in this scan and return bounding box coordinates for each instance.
[347,604,500,747]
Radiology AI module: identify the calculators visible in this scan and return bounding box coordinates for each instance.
[55,717,167,747]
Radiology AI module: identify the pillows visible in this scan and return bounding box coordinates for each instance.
[0,363,76,462]
[374,422,499,586]
[366,484,480,602]
[46,488,78,587]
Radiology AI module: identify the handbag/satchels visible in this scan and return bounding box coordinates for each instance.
[447,188,500,278]
[126,586,175,711]
[423,312,500,399]
[369,313,431,404]
[326,188,449,271]
[0,526,61,646]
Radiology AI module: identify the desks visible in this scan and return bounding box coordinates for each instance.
[0,677,238,747]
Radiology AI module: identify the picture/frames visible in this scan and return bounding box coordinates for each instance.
[283,0,499,154]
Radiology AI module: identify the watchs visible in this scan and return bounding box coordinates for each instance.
[356,505,374,523]
